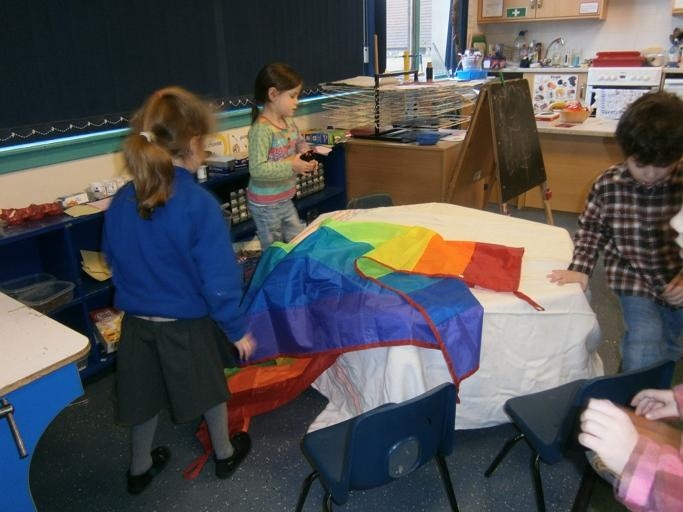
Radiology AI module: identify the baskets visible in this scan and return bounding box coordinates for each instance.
[551,108,591,123]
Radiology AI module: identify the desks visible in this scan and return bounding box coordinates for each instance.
[0,294,92,463]
[288,202,605,433]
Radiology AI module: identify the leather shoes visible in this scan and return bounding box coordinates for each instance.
[126,445,172,493]
[214,430,253,481]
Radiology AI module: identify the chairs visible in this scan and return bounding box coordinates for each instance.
[484,360,675,512]
[345,193,394,208]
[298,381,458,512]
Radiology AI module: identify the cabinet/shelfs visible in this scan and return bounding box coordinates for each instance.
[0,189,125,381]
[474,58,683,214]
[192,142,347,282]
[344,127,526,217]
[479,0,605,23]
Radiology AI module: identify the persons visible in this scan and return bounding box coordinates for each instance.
[102,88,254,495]
[247,63,320,251]
[579,376,683,511]
[546,89,682,372]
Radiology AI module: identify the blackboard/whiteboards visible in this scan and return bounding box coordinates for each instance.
[449,78,493,191]
[487,78,548,204]
[0,0,371,175]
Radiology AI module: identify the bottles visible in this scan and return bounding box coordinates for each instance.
[426,62,433,83]
[669,39,679,68]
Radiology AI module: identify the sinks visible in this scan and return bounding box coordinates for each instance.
[532,66,581,68]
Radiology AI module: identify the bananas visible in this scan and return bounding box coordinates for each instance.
[549,102,568,112]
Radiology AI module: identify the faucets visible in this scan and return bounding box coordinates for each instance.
[540,38,565,65]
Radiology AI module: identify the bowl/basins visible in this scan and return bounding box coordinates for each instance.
[0,271,56,296]
[16,280,76,315]
